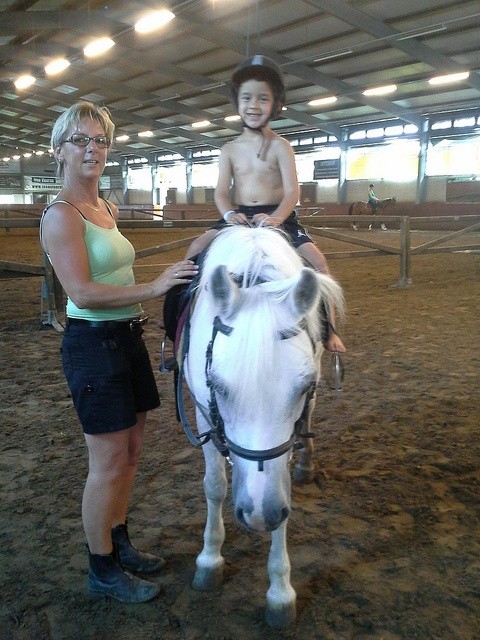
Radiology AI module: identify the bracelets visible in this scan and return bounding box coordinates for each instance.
[223,211,235,221]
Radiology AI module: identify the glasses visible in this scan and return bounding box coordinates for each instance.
[61,134,110,148]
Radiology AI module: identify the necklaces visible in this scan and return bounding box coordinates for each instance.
[65,186,100,211]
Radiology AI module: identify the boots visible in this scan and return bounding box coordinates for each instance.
[85,542,160,604]
[112,524,166,574]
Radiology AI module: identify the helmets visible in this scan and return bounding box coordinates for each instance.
[231,55,285,120]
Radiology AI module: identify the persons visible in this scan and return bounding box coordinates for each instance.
[39,101,199,604]
[185,53,346,353]
[367,184,380,216]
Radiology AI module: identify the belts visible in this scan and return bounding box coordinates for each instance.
[69,314,149,333]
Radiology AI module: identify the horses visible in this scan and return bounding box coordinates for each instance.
[348,196,399,231]
[171,225,344,628]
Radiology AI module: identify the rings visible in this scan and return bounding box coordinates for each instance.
[175,272,178,278]
